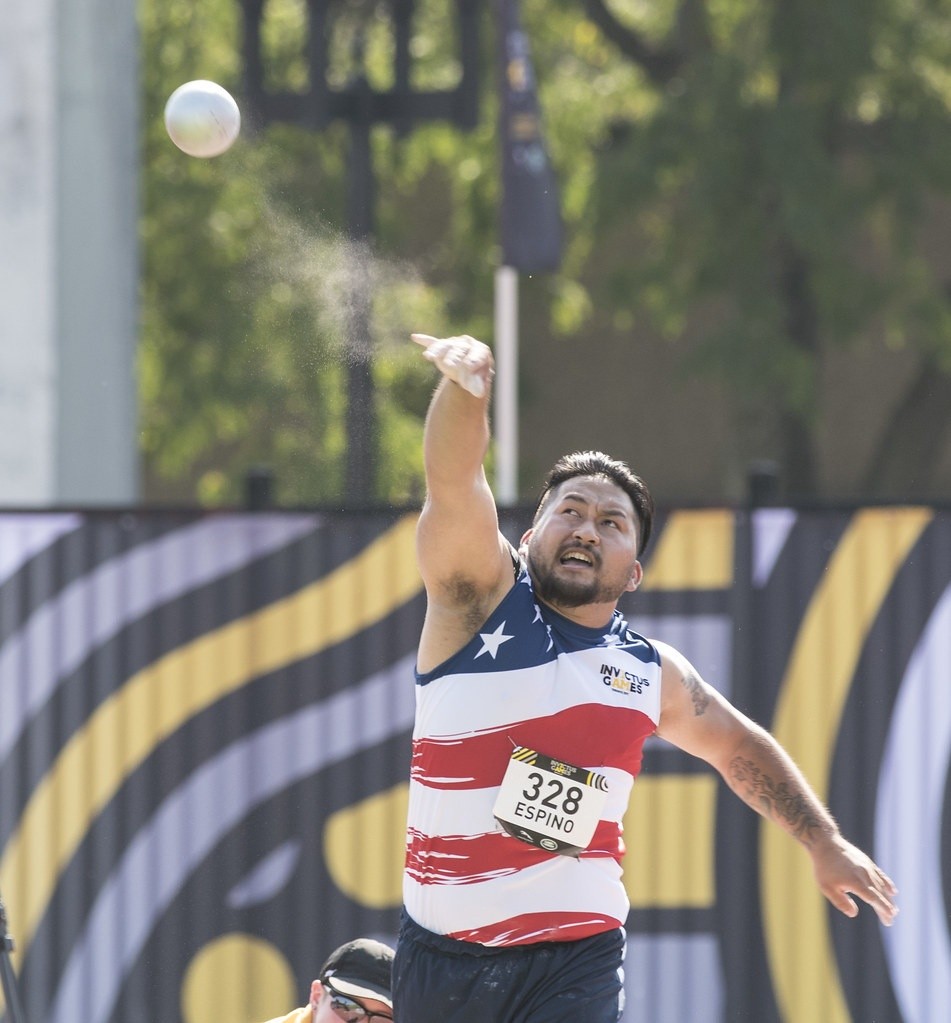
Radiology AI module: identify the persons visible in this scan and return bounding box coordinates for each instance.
[262,939,396,1023]
[392,334,897,1023]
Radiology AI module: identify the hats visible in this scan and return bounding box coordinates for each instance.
[319,938,396,1009]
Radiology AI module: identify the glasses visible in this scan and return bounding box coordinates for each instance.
[323,984,393,1023]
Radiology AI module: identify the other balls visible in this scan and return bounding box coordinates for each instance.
[163,80,240,159]
